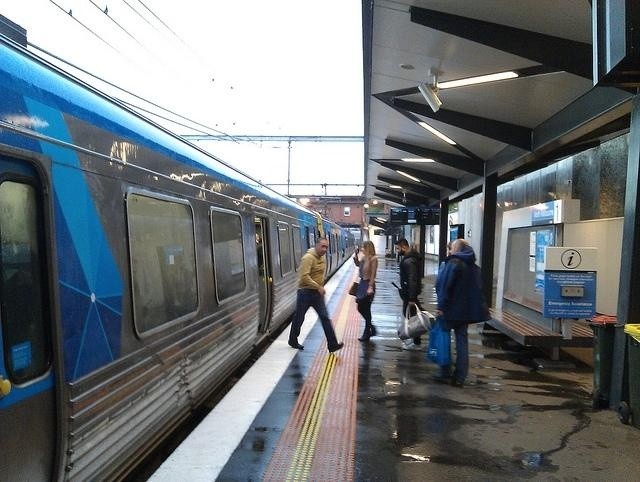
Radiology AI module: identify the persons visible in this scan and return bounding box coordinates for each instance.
[352,240,378,342]
[393,239,422,350]
[434,238,492,389]
[287,238,345,353]
[155,243,198,319]
[434,241,453,298]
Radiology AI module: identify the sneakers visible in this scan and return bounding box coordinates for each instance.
[328,342,343,352]
[435,371,463,387]
[289,342,304,349]
[401,339,422,350]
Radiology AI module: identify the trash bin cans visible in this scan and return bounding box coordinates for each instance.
[588,312,618,409]
[616,323,640,431]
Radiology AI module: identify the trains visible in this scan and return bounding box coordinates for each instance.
[0,12,357,481]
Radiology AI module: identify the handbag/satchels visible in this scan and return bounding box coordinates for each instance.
[398,311,436,340]
[427,330,451,367]
[349,282,359,296]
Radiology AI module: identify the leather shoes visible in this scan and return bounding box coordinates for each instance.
[358,325,376,341]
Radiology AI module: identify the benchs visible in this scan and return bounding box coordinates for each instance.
[484,306,596,369]
